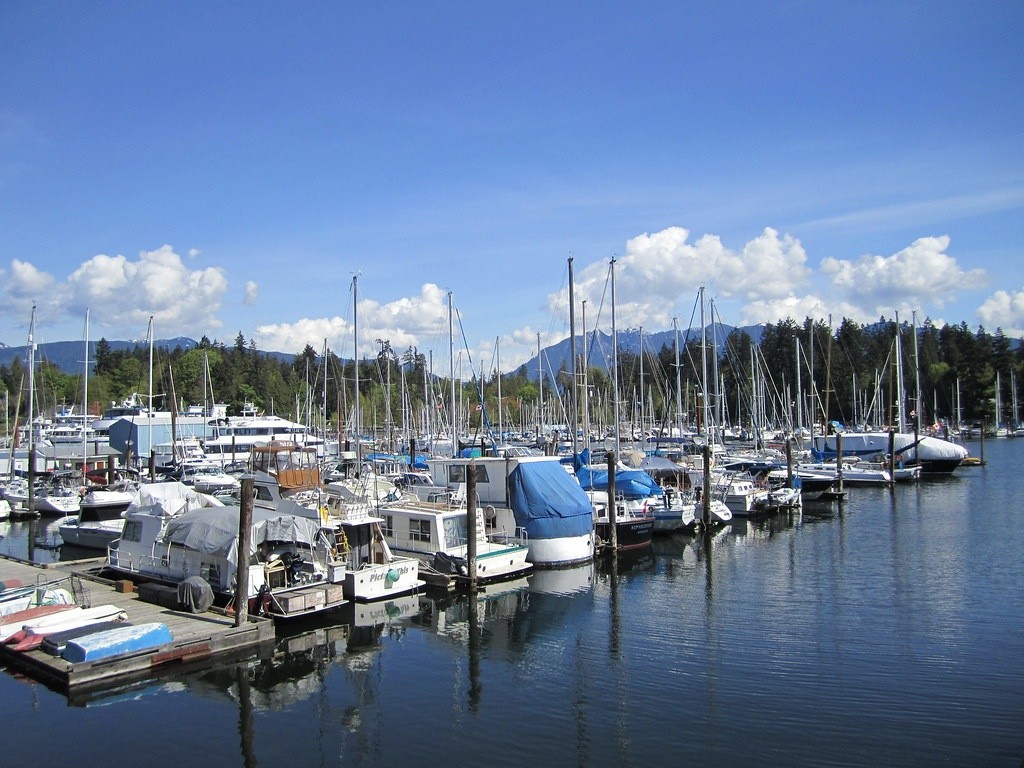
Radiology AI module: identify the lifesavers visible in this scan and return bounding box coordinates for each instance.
[885,453,892,461]
[896,453,904,462]
[881,461,888,470]
[484,504,496,520]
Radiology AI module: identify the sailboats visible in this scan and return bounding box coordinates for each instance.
[1,257,1024,624]
